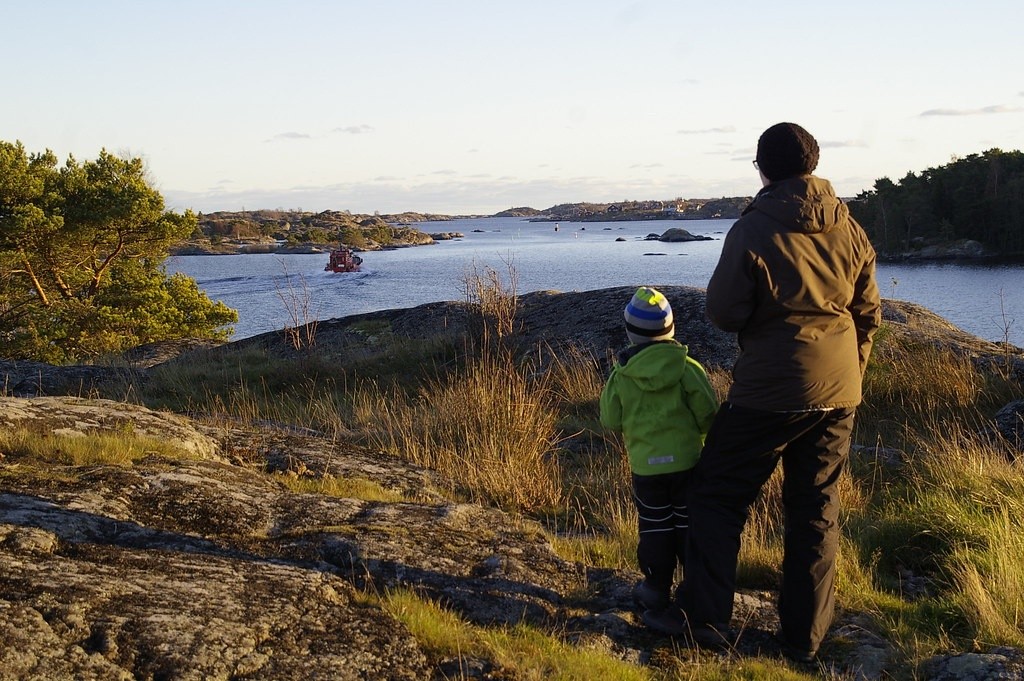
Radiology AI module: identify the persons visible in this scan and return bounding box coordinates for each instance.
[599,287,720,609]
[642,122,881,661]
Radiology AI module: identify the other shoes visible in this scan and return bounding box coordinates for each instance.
[641,608,727,644]
[633,577,671,613]
[776,629,815,663]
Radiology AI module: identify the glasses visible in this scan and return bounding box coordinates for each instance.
[752,159,760,169]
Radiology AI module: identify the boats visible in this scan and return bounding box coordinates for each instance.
[330,249,363,272]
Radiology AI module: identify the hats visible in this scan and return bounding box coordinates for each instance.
[623,286,676,344]
[757,122,820,182]
